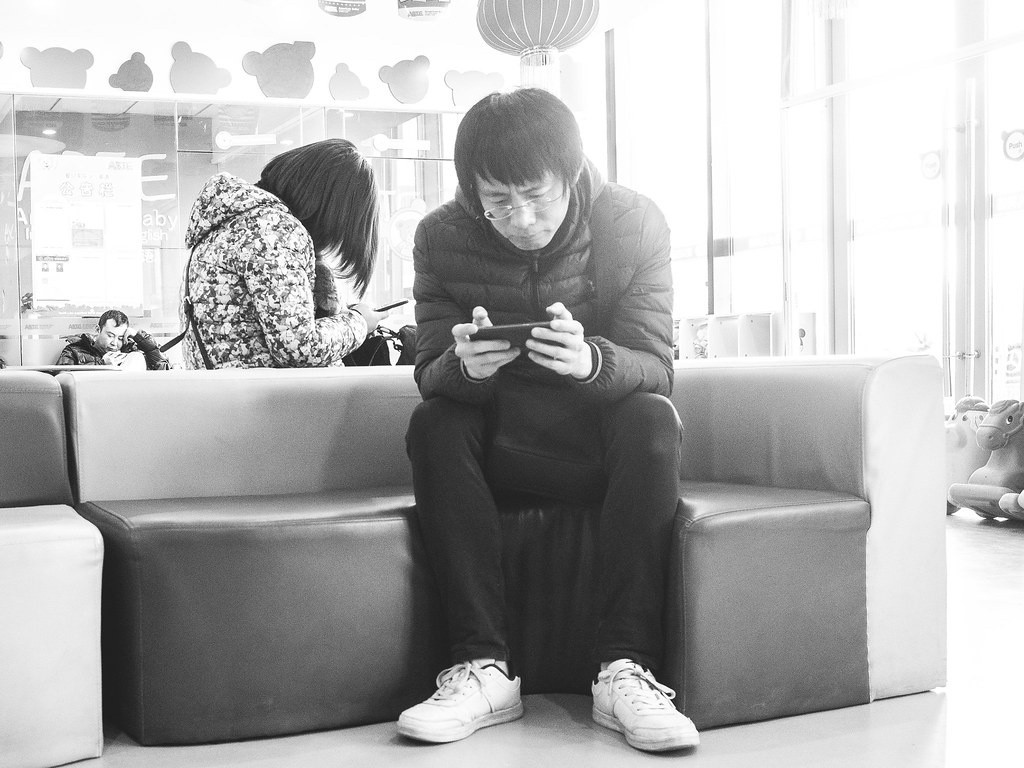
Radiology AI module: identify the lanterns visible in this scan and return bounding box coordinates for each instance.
[476,0,600,88]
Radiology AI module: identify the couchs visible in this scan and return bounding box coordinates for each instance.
[56,347,951,748]
[0,365,104,768]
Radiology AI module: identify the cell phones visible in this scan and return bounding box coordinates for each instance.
[469,321,565,355]
[111,352,128,359]
[374,299,409,312]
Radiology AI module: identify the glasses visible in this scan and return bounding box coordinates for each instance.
[475,179,569,222]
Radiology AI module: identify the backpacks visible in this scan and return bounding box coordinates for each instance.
[367,325,417,366]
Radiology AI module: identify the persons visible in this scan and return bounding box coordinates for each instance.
[56,311,169,374]
[178,138,390,369]
[397,88,701,751]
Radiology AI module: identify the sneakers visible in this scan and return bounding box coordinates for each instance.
[591,658,700,753]
[395,657,524,743]
